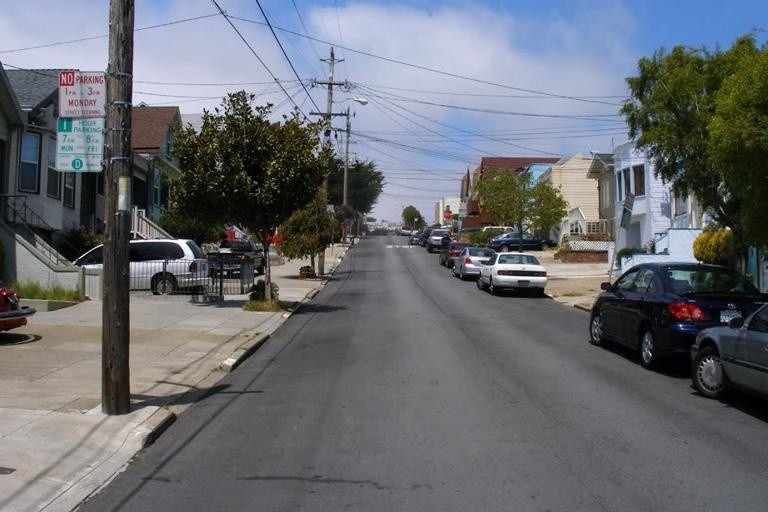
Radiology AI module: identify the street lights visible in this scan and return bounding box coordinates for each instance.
[318,96,368,274]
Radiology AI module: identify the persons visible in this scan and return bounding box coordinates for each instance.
[441,232,452,249]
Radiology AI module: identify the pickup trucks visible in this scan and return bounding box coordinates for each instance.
[206,238,265,280]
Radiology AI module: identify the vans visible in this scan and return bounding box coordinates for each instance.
[68,238,215,299]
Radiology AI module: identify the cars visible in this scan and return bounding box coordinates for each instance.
[685,298,768,404]
[390,223,555,280]
[476,249,547,298]
[1,277,39,342]
[587,256,767,371]
